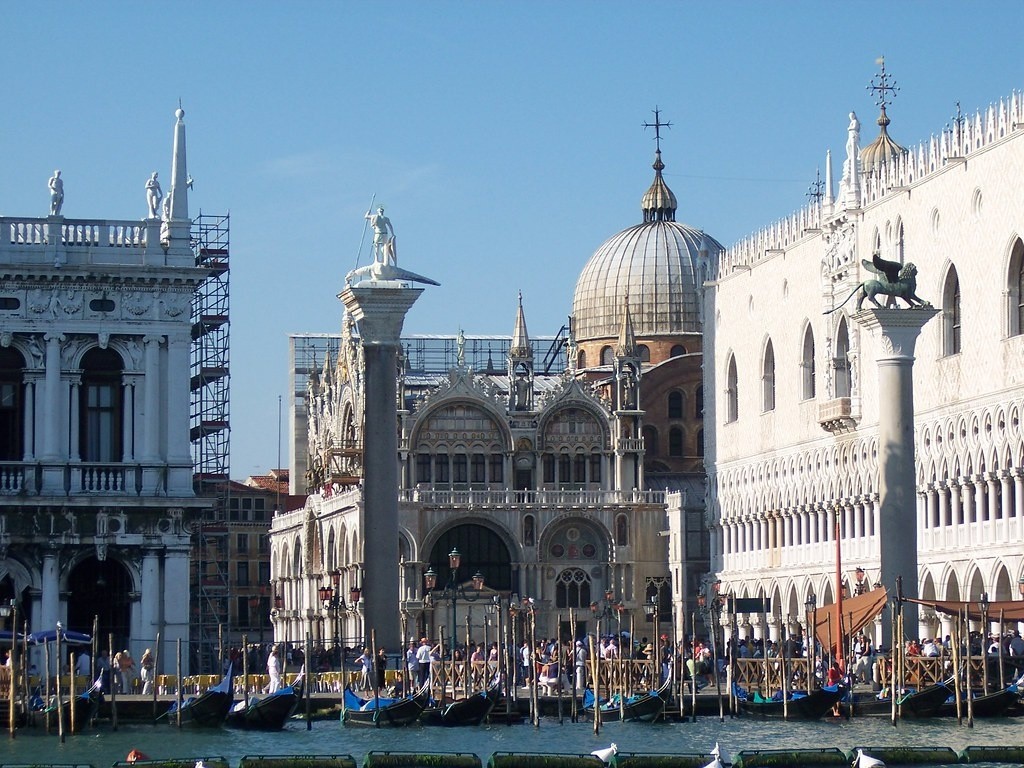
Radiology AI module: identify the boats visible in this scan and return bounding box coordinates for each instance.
[27,667,104,732]
[344,677,430,727]
[937,674,1024,716]
[167,661,234,727]
[421,683,500,726]
[230,664,305,731]
[582,662,672,721]
[732,665,849,721]
[825,662,965,717]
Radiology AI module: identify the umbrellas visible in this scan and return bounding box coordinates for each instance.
[30,628,94,644]
[0,631,35,646]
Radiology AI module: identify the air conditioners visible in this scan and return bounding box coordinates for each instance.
[157,517,174,535]
[105,515,126,533]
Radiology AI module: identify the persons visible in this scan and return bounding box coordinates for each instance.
[568,335,578,359]
[406,637,507,695]
[96,650,135,695]
[365,208,395,265]
[828,662,849,717]
[163,191,171,219]
[48,170,64,215]
[846,112,860,159]
[509,629,1024,694]
[145,172,162,218]
[62,648,91,695]
[140,648,154,695]
[231,648,240,675]
[262,646,281,694]
[27,665,45,689]
[457,329,466,357]
[5,650,9,666]
[355,647,403,698]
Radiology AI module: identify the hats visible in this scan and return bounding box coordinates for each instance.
[419,638,428,643]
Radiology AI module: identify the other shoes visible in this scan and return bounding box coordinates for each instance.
[710,684,715,687]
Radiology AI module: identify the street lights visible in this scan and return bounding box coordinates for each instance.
[424,546,484,661]
[641,595,657,686]
[590,589,625,638]
[804,593,817,690]
[0,597,16,695]
[248,581,281,674]
[485,595,501,682]
[697,578,728,659]
[509,595,537,645]
[318,569,361,671]
[840,569,881,636]
